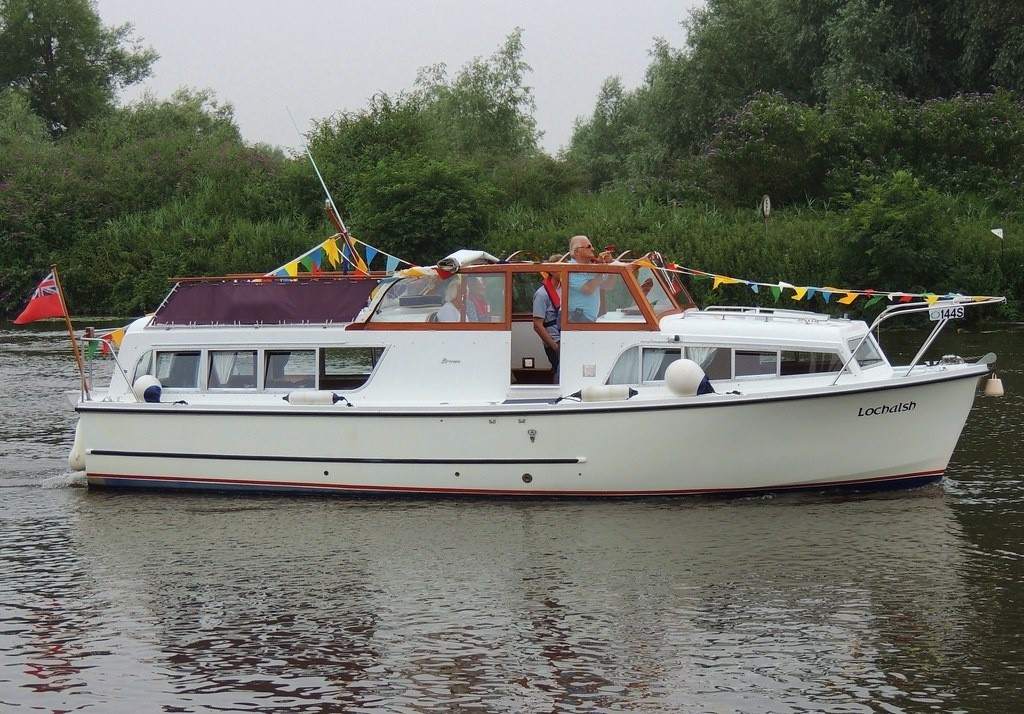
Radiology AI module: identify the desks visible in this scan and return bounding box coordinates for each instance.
[272,375,309,387]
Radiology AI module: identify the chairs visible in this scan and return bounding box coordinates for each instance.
[428,311,438,322]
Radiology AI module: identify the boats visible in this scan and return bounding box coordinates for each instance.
[68,257,1007,501]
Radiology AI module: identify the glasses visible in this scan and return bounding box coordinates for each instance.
[576,244,595,249]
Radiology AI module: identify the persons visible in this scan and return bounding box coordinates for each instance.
[532,255,569,380]
[553,234,616,385]
[437,275,489,324]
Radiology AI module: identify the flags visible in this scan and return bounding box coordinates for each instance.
[14,271,66,327]
